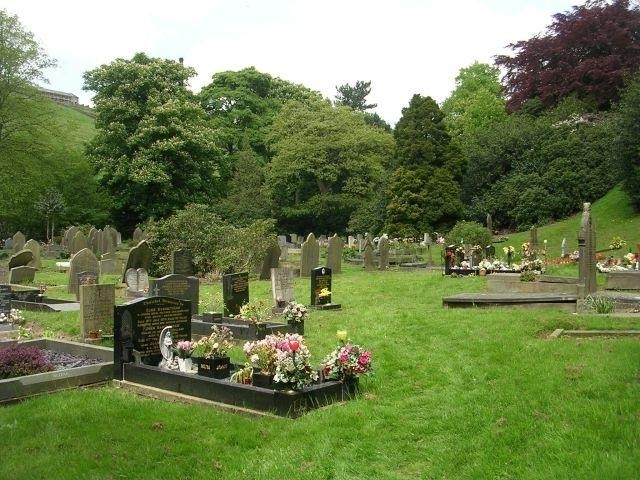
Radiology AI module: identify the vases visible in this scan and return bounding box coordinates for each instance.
[248,322,267,340]
[196,354,231,380]
[320,297,327,305]
[177,357,194,373]
[288,319,304,335]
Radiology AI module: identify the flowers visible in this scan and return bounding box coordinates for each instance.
[320,328,375,381]
[9,307,24,326]
[435,236,547,272]
[230,300,272,324]
[283,301,307,325]
[195,323,235,358]
[603,250,638,273]
[244,331,318,390]
[318,287,332,304]
[174,340,193,358]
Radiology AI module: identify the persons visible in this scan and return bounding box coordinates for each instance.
[159,331,178,371]
